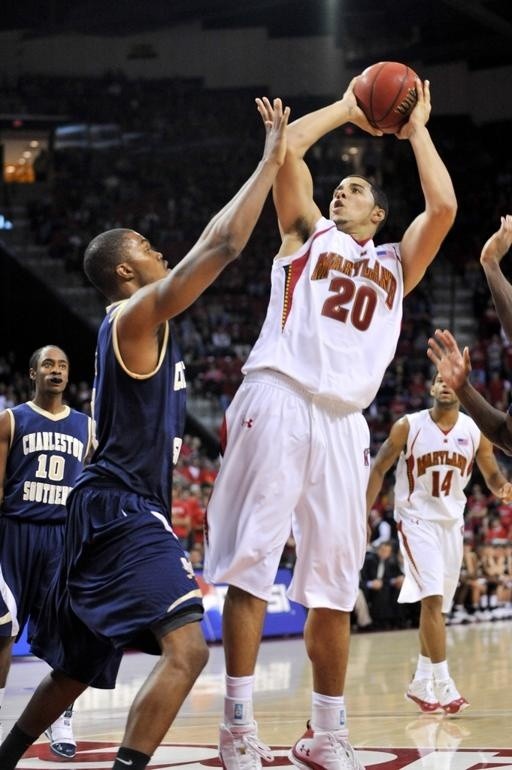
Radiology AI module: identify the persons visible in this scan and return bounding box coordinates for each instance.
[204,70,460,769]
[0,88,290,770]
[0,341,103,763]
[1,74,512,628]
[365,362,511,719]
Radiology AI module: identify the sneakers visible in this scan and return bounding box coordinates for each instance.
[218,719,264,770]
[443,602,512,626]
[404,679,441,714]
[287,719,365,770]
[433,678,471,716]
[44,701,77,758]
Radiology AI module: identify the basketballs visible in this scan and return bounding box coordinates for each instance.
[356,61,424,137]
